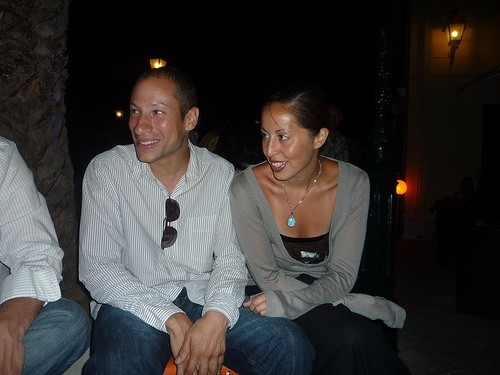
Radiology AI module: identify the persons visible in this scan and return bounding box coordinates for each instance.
[0,136,92,375]
[79,64,315,375]
[188,85,415,375]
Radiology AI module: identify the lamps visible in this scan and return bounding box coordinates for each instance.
[441,9,474,66]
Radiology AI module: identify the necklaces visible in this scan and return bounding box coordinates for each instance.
[282,158,322,229]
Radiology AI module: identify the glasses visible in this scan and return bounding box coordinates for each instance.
[161,196,180,250]
